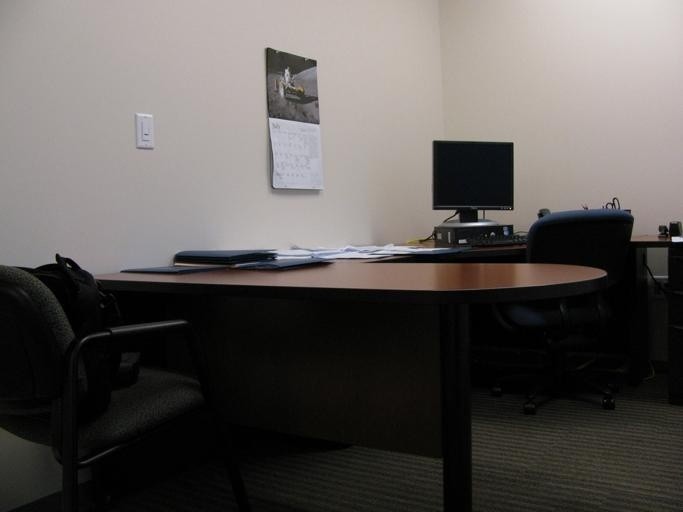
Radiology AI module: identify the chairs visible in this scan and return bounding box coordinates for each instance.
[1,259,256,510]
[489,208,634,415]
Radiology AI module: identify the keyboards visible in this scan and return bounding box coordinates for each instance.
[470,235,529,246]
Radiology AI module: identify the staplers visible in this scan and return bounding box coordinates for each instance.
[659,226,668,238]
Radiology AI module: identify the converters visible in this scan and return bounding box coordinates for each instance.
[669,221,682,236]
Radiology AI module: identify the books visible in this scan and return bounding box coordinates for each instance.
[119,246,332,277]
[375,243,469,258]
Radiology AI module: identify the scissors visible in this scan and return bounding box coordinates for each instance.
[606,197,620,210]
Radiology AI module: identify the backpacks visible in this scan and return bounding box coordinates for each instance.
[11,252,126,424]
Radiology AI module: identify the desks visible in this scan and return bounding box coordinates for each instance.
[93,232,682,511]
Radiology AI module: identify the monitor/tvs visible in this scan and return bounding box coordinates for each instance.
[432,140,514,228]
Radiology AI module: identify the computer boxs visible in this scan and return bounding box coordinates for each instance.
[434,225,514,250]
